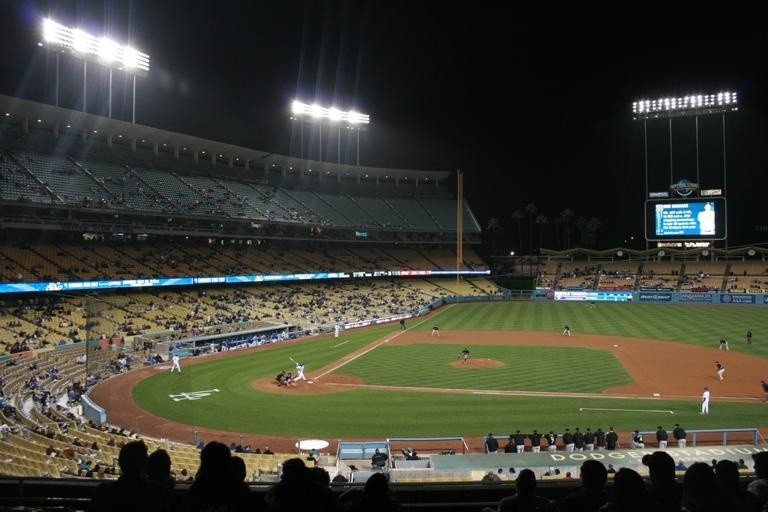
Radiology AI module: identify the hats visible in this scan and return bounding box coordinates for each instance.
[640,449,676,479]
[148,448,175,466]
[364,470,397,500]
[279,458,309,488]
[681,461,715,490]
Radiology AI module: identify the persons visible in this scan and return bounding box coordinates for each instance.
[2,155,768,365]
[2,356,768,511]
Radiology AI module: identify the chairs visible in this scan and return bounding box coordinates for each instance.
[542,255,768,296]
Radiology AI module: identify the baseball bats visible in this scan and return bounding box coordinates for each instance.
[288,357,297,366]
